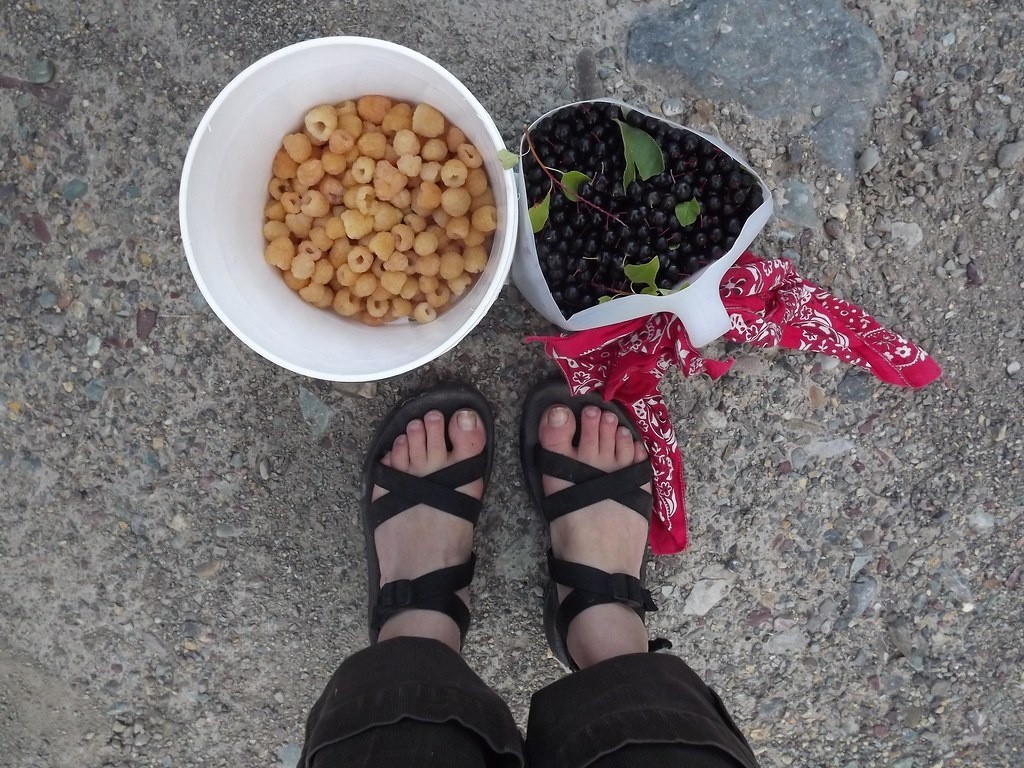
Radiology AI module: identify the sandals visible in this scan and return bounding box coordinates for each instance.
[359,384,496,659]
[522,381,674,678]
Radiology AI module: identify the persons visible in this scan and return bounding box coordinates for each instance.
[294,381,757,768]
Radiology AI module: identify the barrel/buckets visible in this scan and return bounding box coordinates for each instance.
[177,37,518,383]
